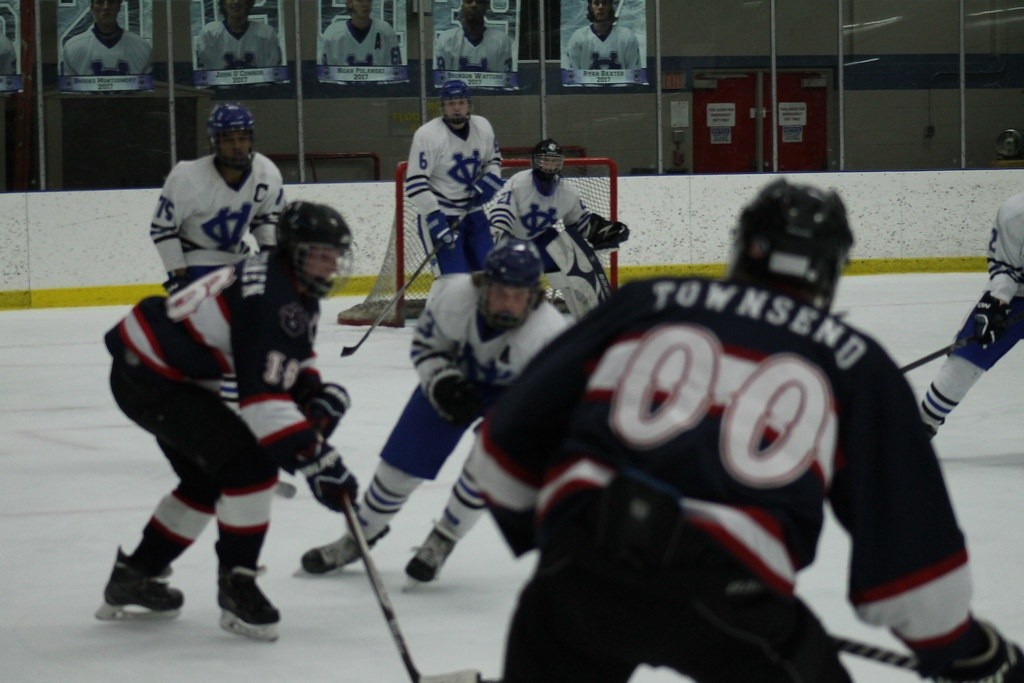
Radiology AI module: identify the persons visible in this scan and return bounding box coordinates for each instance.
[918,190,1024,439]
[63,0,159,75]
[471,177,1024,683]
[197,0,282,69]
[93,202,359,642]
[436,0,512,73]
[321,0,402,67]
[290,239,565,596]
[567,0,641,74]
[149,101,287,412]
[487,139,629,322]
[405,80,504,279]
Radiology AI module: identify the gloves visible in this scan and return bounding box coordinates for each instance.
[587,211,629,250]
[302,448,359,513]
[435,376,480,420]
[975,290,1012,350]
[469,174,505,208]
[440,231,460,250]
[890,619,1023,683]
[300,384,347,441]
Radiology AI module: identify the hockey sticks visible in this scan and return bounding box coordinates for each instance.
[897,319,1006,374]
[336,487,486,683]
[341,196,477,359]
[828,625,938,673]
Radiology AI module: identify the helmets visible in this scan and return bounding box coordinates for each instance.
[440,80,472,125]
[532,139,564,182]
[275,202,353,296]
[207,99,255,166]
[482,246,539,329]
[727,180,854,310]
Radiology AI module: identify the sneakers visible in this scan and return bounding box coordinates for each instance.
[302,524,390,573]
[96,547,183,622]
[218,564,281,640]
[405,524,456,582]
